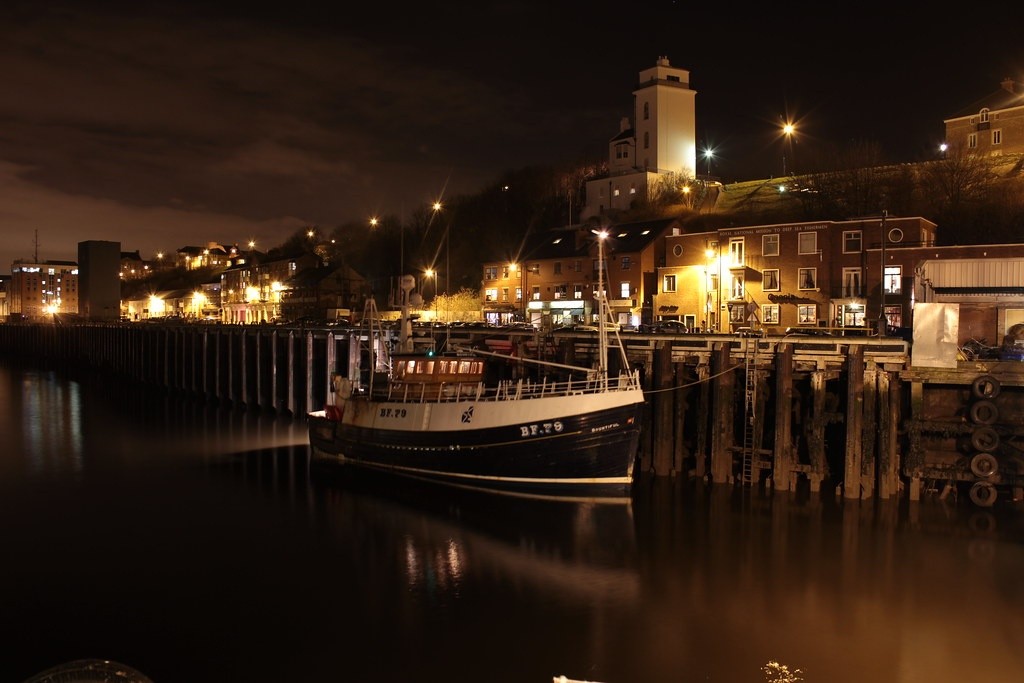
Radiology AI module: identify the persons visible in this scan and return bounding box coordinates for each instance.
[1000,335,1015,353]
[701,321,705,333]
[804,318,807,322]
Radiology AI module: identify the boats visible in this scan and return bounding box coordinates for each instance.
[307,228,645,511]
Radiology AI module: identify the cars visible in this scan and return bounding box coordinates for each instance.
[573,321,616,332]
[640,320,689,333]
[735,326,764,335]
[274,317,532,328]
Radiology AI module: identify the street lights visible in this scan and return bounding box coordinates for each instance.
[508,263,522,321]
[704,249,716,334]
[783,122,794,176]
[704,148,713,220]
[425,269,437,321]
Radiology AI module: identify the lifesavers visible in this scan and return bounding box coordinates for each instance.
[969,480,997,507]
[971,427,1000,452]
[970,453,998,478]
[972,375,1000,400]
[971,400,1000,425]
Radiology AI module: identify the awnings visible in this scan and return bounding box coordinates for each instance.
[570,309,585,315]
[550,309,563,314]
